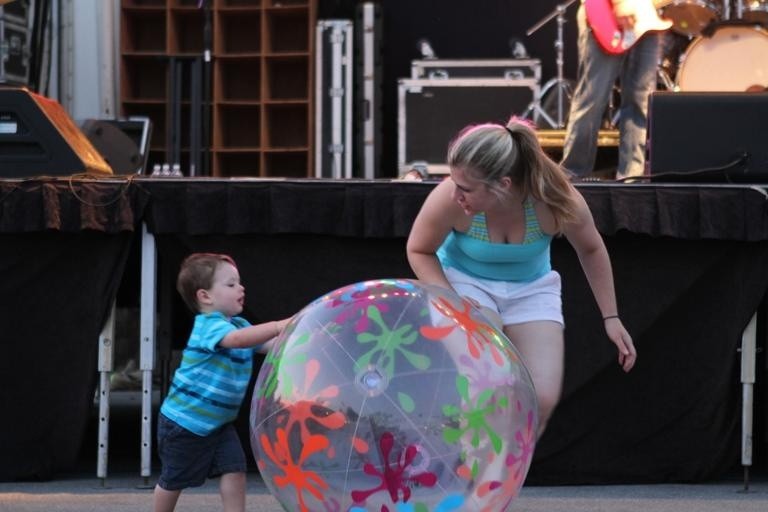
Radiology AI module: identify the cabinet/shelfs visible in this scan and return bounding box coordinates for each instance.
[118,0,319,183]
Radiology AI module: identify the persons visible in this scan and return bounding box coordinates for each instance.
[555,0,667,184]
[405,115,639,512]
[153,252,298,512]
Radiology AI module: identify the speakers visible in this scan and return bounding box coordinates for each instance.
[73,118,145,175]
[1,84,115,180]
[643,91,768,188]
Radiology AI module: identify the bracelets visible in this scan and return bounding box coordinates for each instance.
[602,315,620,322]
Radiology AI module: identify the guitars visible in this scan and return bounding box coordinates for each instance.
[586,0,675,57]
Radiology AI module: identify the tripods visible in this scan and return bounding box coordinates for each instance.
[522,10,581,131]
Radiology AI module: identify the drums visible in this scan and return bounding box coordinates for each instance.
[674,20,767,91]
[737,0,767,22]
[662,0,723,36]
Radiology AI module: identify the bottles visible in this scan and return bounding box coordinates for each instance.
[150,164,184,177]
